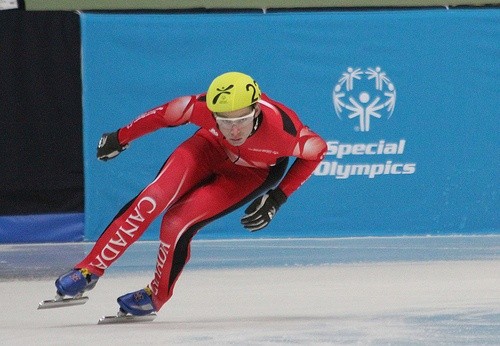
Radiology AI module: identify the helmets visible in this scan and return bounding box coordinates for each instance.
[206,72,261,112]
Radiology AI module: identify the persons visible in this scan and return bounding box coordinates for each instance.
[37,71,328,325]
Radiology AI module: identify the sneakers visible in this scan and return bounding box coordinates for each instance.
[39,268,99,309]
[99,285,157,324]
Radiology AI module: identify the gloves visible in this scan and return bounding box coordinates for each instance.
[96,127,128,161]
[241,186,287,232]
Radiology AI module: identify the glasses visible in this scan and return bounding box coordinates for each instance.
[213,106,255,129]
